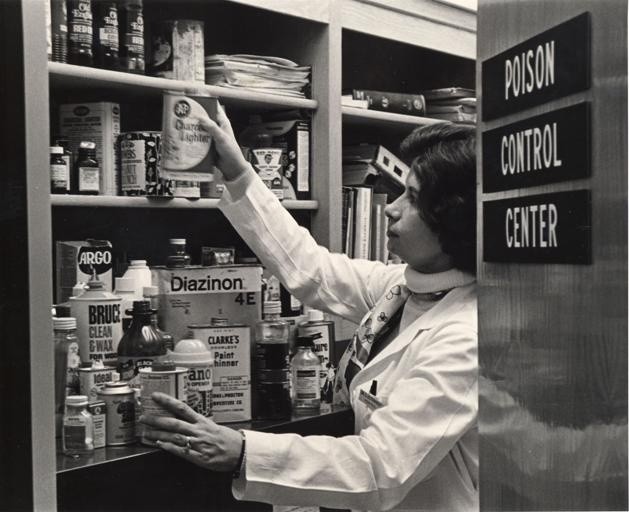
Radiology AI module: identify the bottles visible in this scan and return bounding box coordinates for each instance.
[252,298,340,416]
[52,258,213,454]
[238,113,273,173]
[50,138,101,194]
[150,14,174,78]
[50,0,146,74]
[165,237,193,269]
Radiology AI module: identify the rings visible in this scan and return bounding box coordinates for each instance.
[183,435,192,451]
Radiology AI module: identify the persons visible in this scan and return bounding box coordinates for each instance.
[136,86,479,511]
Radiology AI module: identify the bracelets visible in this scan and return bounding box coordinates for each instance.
[229,432,245,481]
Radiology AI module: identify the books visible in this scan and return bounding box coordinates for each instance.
[204,51,477,125]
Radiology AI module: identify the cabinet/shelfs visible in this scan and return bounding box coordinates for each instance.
[17,2,482,512]
